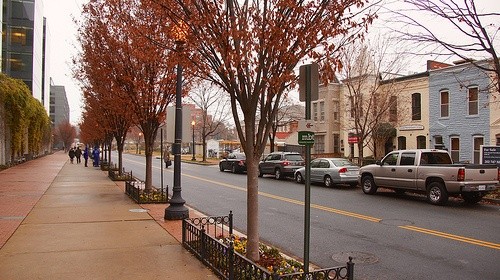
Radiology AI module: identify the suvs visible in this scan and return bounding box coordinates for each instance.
[258,151,305,180]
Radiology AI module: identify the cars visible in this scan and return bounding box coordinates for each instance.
[292,157,361,188]
[219,147,247,174]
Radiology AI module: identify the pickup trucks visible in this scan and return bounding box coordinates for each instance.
[356,148,500,206]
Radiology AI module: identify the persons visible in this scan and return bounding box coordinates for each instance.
[68,147,82,163]
[84,147,90,167]
[92,146,100,167]
[164,150,169,168]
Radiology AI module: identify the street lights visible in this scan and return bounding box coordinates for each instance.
[163,18,189,221]
[192,121,196,160]
[138,132,142,154]
